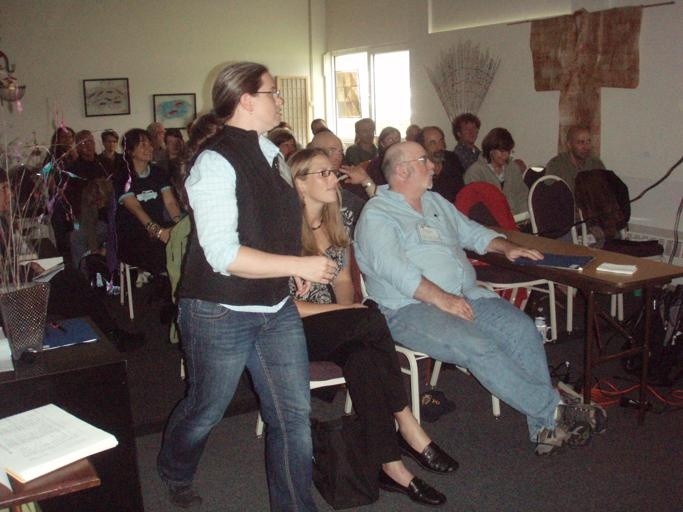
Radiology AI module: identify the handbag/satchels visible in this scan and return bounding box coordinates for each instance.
[311,415,378,509]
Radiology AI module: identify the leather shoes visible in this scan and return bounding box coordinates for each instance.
[170,487,201,508]
[397,429,459,475]
[381,469,446,505]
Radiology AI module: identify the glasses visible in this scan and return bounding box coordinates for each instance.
[303,170,330,177]
[257,89,280,96]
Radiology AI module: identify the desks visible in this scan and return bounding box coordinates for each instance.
[463,222,683,426]
[0,314,145,511]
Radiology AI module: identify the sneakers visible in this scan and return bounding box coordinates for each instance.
[536,402,607,456]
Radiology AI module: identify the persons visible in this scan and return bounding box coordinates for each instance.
[0,167,149,352]
[154,58,340,512]
[35,116,629,511]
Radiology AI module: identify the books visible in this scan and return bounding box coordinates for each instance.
[1,403,121,486]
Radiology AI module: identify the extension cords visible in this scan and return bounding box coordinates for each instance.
[556,380,595,407]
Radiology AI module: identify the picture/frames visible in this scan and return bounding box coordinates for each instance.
[82,77,131,118]
[152,92,197,131]
